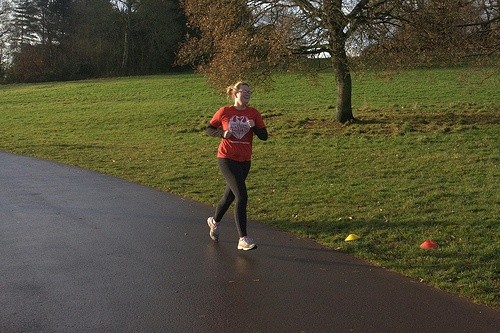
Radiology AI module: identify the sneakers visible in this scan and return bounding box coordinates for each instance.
[207,217,219,241]
[237,236,257,250]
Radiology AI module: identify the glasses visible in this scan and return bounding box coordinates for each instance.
[237,90,252,95]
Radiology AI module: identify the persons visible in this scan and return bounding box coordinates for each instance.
[205,80,269,251]
